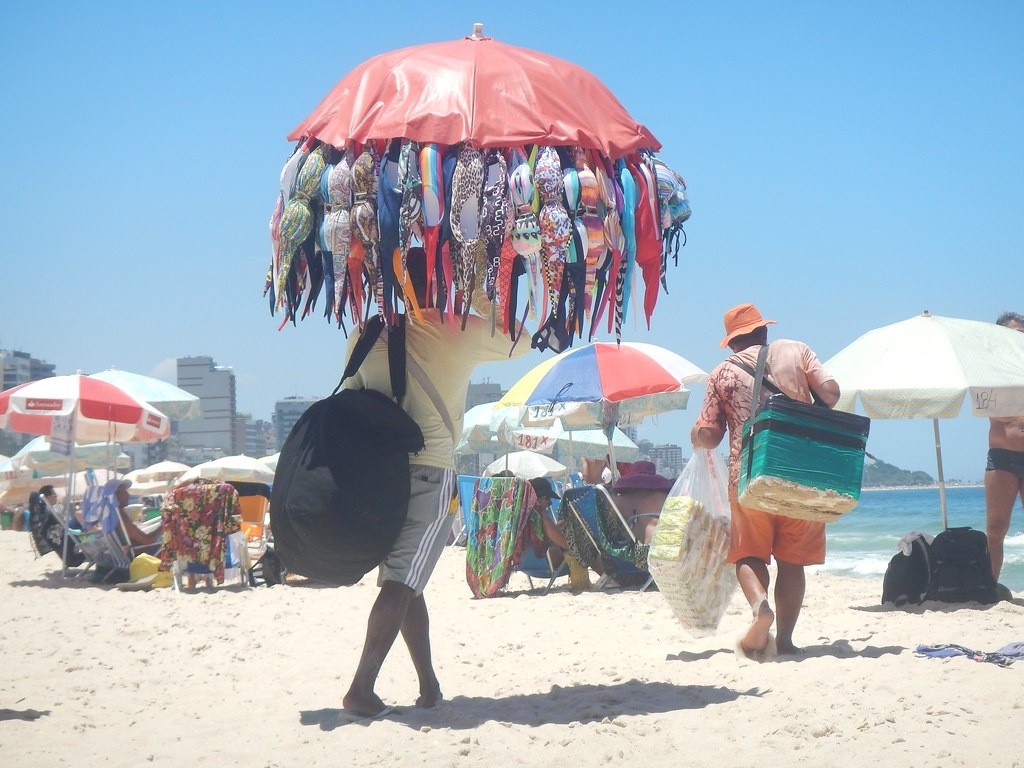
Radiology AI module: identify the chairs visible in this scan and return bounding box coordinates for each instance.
[29,481,287,591]
[458,475,659,596]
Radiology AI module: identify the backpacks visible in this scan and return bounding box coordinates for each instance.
[269,315,425,585]
[881,526,998,605]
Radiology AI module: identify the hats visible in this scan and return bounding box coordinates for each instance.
[528,477,562,499]
[720,303,778,348]
[612,461,673,489]
[104,479,132,494]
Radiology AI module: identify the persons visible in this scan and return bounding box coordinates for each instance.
[343,247,531,719]
[39,485,100,531]
[530,477,569,551]
[488,338,710,485]
[103,479,163,544]
[984,312,1024,584]
[690,303,840,657]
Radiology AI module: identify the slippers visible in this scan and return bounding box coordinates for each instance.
[338,706,394,721]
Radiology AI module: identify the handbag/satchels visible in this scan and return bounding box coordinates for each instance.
[647,448,739,640]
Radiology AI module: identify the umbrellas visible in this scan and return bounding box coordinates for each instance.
[454,401,639,487]
[287,23,662,160]
[809,310,1024,531]
[0,366,282,580]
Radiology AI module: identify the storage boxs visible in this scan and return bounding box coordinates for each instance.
[738,399,869,526]
